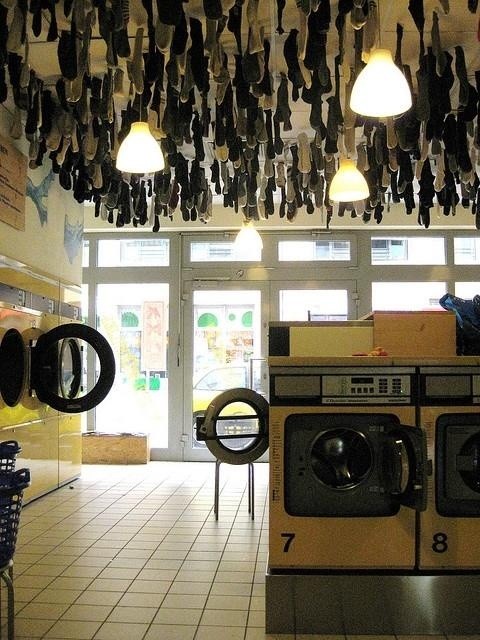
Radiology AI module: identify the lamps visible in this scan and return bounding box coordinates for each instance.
[349,1,413,117]
[229,214,266,251]
[115,14,165,174]
[329,65,371,204]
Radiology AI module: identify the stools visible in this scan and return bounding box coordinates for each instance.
[211,456,255,519]
[1,561,19,639]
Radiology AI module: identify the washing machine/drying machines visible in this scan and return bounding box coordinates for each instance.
[418,360,480,571]
[207,356,415,575]
[0,255,116,516]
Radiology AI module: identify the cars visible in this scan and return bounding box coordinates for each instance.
[195,364,265,448]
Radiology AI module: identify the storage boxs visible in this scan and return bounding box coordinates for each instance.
[374,313,458,357]
[287,326,375,359]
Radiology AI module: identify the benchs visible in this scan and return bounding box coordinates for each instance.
[82,432,149,465]
